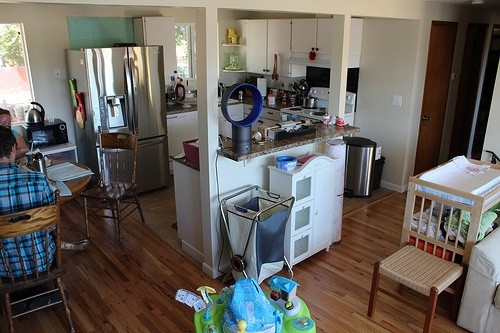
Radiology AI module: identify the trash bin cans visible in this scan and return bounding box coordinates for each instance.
[343,136,377,198]
[373,155,385,191]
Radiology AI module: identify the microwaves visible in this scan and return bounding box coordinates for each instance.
[22,119,69,151]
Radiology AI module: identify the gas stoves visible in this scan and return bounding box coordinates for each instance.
[280,87,356,121]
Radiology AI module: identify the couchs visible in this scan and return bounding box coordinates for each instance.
[457,226,500,333]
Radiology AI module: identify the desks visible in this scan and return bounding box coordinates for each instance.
[42,142,78,163]
[48,160,92,203]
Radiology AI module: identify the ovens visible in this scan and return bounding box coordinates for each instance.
[282,113,322,124]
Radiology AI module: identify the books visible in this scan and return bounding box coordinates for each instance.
[47,162,94,182]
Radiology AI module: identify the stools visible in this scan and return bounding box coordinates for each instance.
[367,242,467,333]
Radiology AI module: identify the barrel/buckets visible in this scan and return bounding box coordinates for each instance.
[343,136,377,198]
[371,156,385,190]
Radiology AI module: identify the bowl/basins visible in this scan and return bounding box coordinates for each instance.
[326,139,346,159]
[276,156,297,171]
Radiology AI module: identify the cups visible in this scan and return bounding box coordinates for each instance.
[48,113,54,122]
[25,155,33,169]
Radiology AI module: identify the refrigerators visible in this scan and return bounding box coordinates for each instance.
[66,46,170,199]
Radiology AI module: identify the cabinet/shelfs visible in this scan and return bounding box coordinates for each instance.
[221,18,363,78]
[244,105,281,131]
[266,155,339,268]
[166,104,243,166]
[400,158,500,297]
[133,15,178,86]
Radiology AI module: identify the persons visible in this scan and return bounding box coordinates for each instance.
[0,108,27,160]
[0,125,71,317]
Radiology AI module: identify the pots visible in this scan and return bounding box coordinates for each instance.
[302,95,318,109]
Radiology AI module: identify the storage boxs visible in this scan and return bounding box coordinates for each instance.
[182,138,199,164]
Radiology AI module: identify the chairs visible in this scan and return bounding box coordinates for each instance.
[77,124,145,245]
[0,188,75,333]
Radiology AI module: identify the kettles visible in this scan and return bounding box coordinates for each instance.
[25,102,45,127]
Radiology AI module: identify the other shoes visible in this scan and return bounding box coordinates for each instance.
[30,289,69,311]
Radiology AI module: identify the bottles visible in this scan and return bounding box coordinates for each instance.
[166,71,180,104]
[34,152,46,174]
[228,29,238,45]
[323,114,344,126]
[268,88,296,107]
